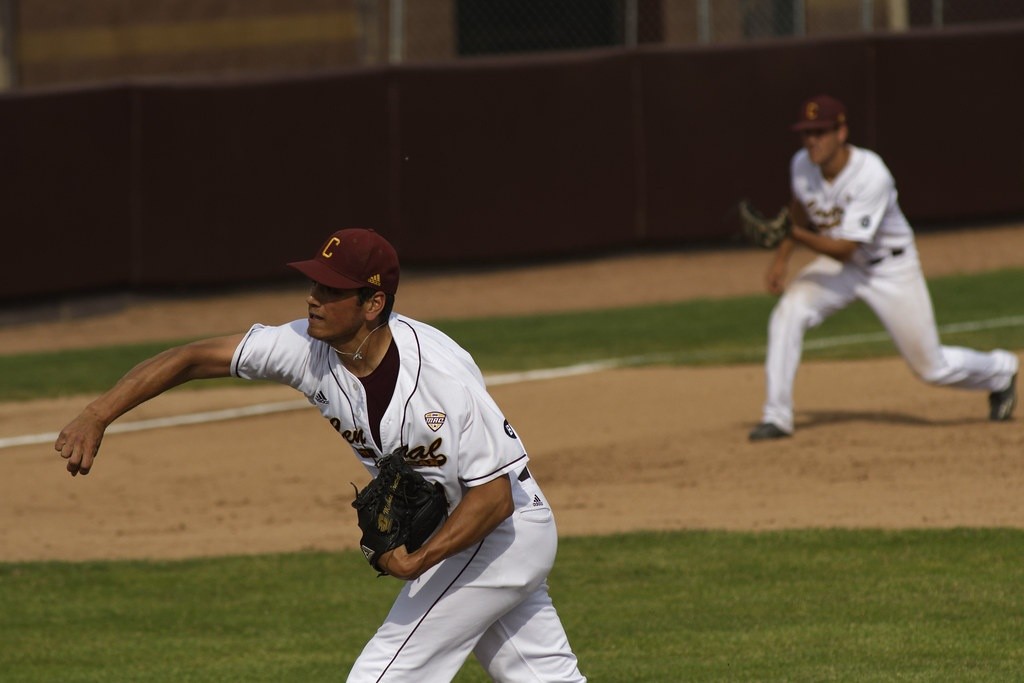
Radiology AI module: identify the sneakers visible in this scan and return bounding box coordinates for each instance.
[747,422,787,440]
[989,372,1016,422]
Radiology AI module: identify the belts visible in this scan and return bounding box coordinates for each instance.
[862,249,903,267]
[517,465,530,482]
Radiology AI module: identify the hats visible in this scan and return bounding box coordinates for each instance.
[287,228,400,294]
[790,95,846,130]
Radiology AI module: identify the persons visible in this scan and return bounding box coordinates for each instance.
[56,228,588,683]
[748,95,1020,441]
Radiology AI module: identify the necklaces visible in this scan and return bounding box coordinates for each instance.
[331,321,388,360]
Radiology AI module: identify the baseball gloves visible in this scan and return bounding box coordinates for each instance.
[737,200,790,248]
[350,443,450,579]
